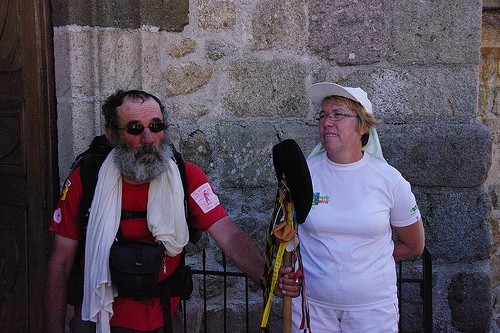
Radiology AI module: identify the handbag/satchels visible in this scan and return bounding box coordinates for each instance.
[109,240,182,300]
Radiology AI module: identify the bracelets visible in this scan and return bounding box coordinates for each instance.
[260,275,264,288]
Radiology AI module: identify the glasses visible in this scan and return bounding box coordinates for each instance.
[314,112,359,123]
[112,120,165,135]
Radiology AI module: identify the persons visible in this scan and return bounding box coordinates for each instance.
[46,90,301,333]
[291,81,424,333]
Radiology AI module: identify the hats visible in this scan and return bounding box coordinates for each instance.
[306,82,372,115]
